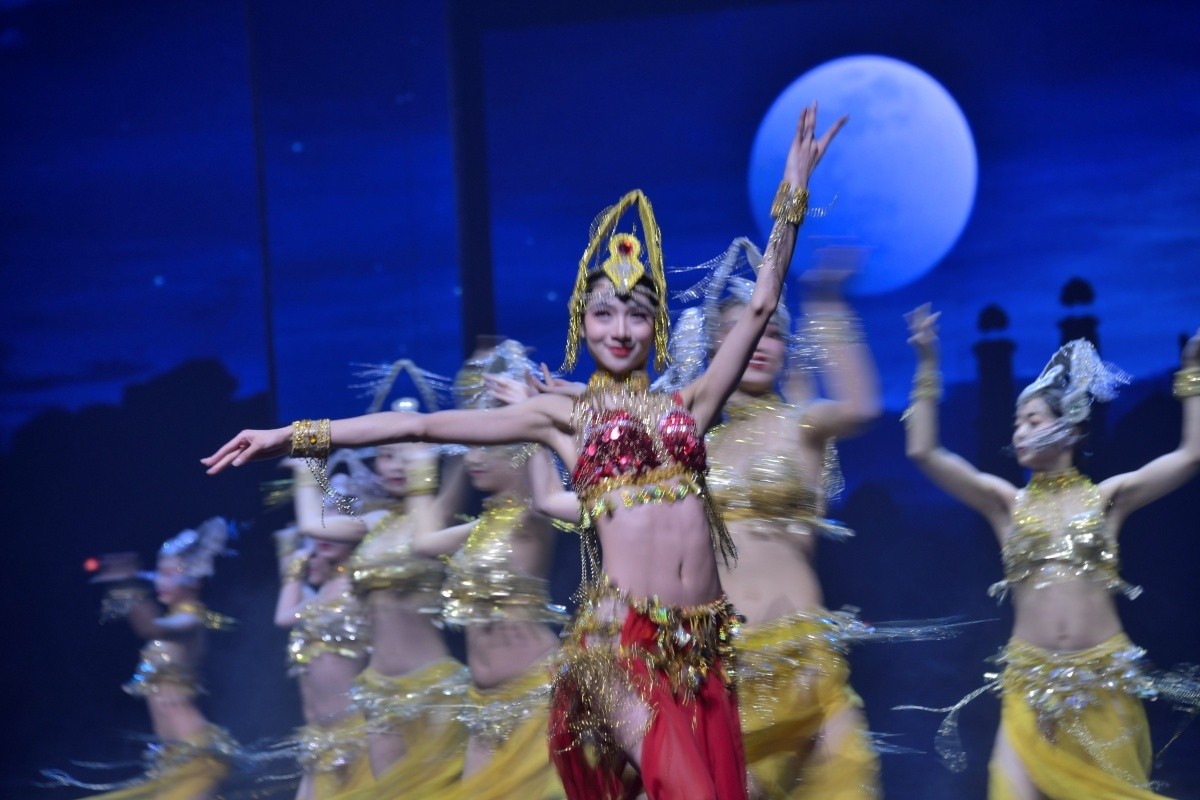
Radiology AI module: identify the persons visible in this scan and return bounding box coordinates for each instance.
[199,99,852,799]
[409,340,577,799]
[272,361,468,800]
[476,235,884,799]
[85,518,244,800]
[273,444,382,800]
[902,303,1200,800]
